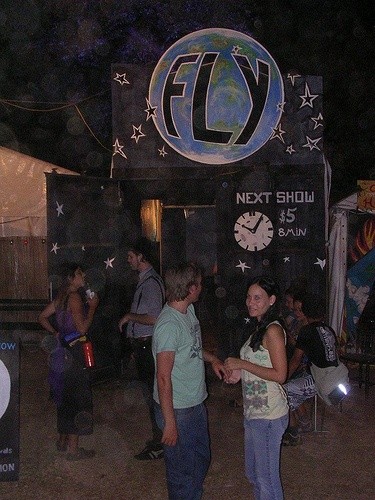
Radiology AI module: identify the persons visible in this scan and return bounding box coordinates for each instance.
[37,262,99,460]
[117,243,166,460]
[222,275,285,500]
[152,260,231,500]
[281,284,339,446]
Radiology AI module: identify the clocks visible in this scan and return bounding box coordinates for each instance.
[233,211,275,253]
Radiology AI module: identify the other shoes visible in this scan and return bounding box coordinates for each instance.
[56,441,95,461]
[134,450,164,461]
[281,433,303,446]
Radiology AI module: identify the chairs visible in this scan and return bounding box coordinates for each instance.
[342,319,375,397]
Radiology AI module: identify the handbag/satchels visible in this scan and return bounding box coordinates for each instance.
[131,337,152,359]
